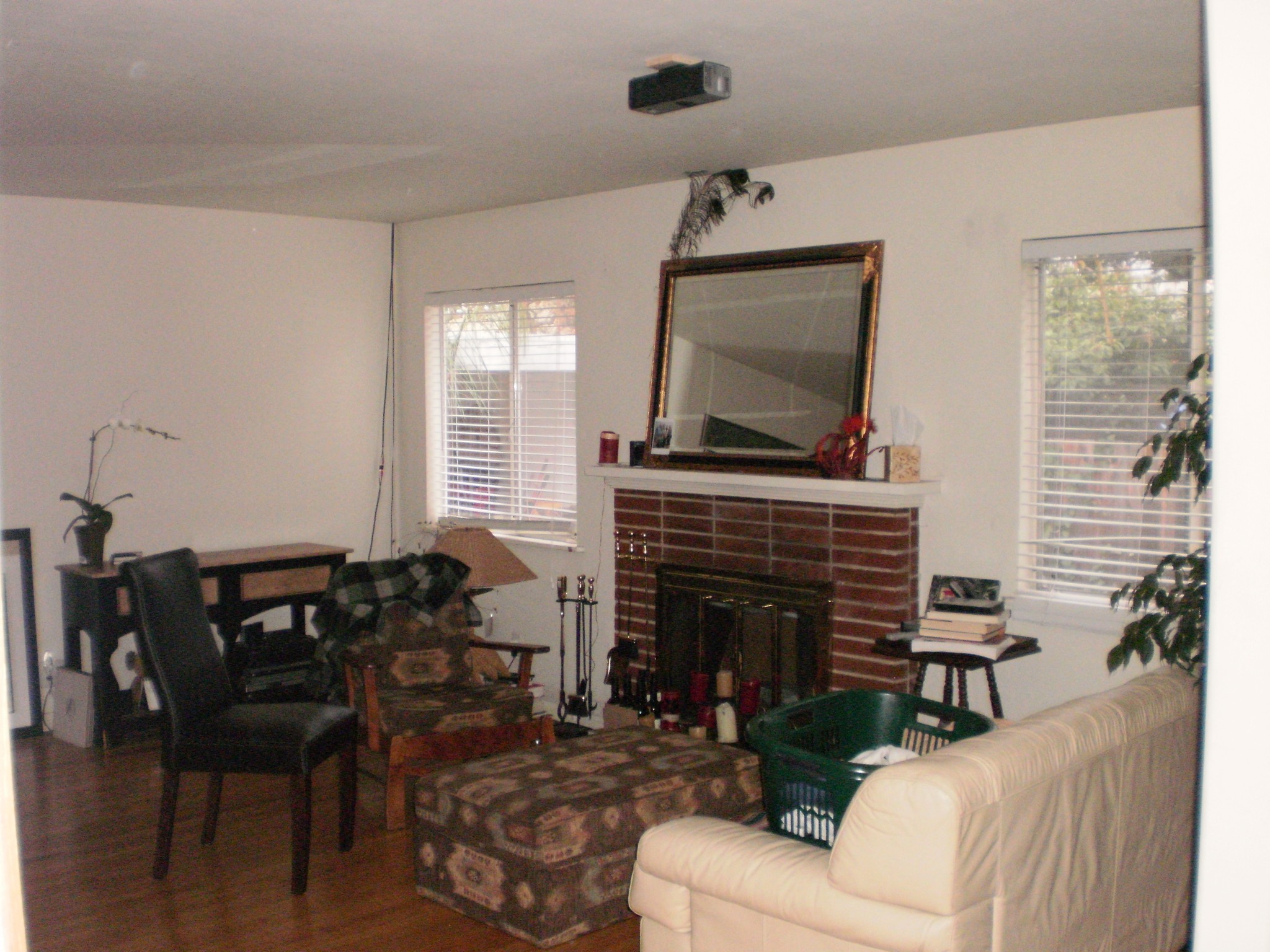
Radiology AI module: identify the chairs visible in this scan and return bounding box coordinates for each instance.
[327,553,550,831]
[120,547,360,894]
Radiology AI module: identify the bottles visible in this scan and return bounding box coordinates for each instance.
[608,670,658,730]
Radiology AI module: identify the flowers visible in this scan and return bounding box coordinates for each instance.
[57,411,180,542]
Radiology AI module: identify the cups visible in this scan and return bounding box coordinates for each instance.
[629,441,644,466]
[662,671,762,743]
[599,431,620,465]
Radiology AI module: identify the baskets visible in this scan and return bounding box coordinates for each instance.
[746,686,997,849]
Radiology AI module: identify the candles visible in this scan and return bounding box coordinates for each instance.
[689,726,706,739]
[717,670,733,696]
[716,703,738,743]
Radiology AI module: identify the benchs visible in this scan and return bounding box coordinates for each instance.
[415,723,769,949]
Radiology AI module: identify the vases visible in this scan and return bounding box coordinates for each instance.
[73,526,104,562]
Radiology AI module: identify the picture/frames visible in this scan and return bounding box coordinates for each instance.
[0,526,46,743]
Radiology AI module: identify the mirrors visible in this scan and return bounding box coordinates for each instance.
[644,239,883,480]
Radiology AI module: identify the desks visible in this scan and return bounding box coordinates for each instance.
[871,633,1043,719]
[54,543,353,747]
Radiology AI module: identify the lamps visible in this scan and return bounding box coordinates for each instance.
[426,527,538,600]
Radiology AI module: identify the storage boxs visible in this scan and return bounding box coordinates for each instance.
[748,688,997,847]
[885,447,920,482]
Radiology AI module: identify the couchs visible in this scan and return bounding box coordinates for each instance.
[627,663,1200,952]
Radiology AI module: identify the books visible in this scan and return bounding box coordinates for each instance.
[910,596,1015,661]
